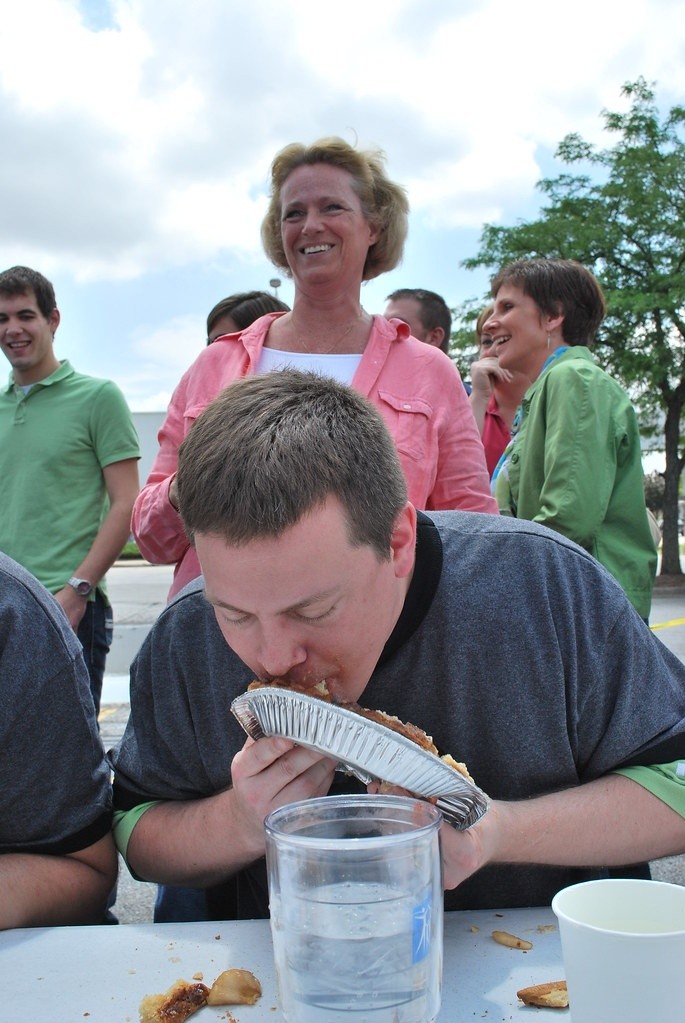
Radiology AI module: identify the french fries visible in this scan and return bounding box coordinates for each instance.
[492,930,533,949]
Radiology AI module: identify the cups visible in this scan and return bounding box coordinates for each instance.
[263,794,444,1023]
[551,879,685,1023]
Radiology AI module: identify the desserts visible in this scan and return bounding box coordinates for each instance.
[139,968,261,1023]
[516,981,568,1008]
[243,677,489,832]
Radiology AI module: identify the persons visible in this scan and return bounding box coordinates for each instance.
[114,367,684,924]
[131,135,657,630]
[0,548,119,930]
[0,265,140,735]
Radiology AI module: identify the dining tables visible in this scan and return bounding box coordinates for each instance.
[0,906,573,1023]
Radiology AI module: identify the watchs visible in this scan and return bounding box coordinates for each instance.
[66,577,91,597]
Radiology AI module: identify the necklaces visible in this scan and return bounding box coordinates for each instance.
[290,311,364,355]
